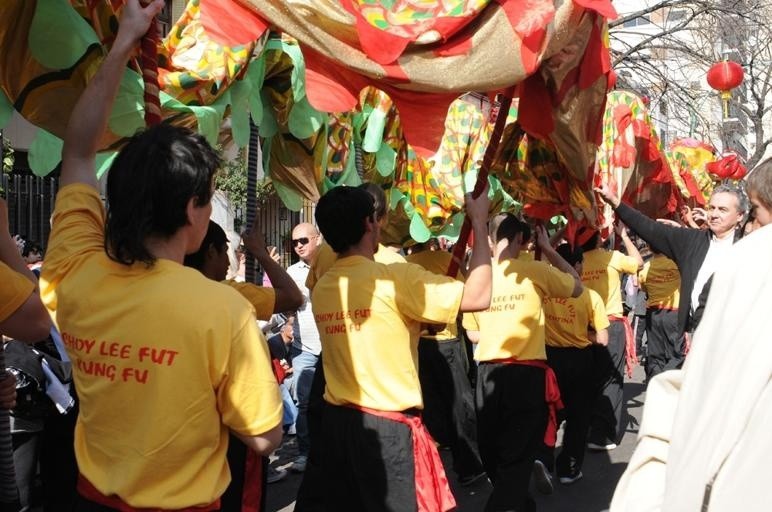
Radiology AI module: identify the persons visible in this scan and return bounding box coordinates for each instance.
[461,213,582,510]
[40,0,284,509]
[0,199,76,511]
[535,162,772,511]
[185,181,496,512]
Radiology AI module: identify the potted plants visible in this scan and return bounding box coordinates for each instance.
[281,229,292,253]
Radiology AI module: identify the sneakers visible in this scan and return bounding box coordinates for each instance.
[558,469,584,485]
[587,436,617,451]
[532,459,554,496]
[290,456,308,472]
[457,469,488,486]
[265,463,288,484]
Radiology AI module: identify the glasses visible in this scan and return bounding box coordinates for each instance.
[291,236,316,248]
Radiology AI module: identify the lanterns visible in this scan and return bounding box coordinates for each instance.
[707,58,743,119]
